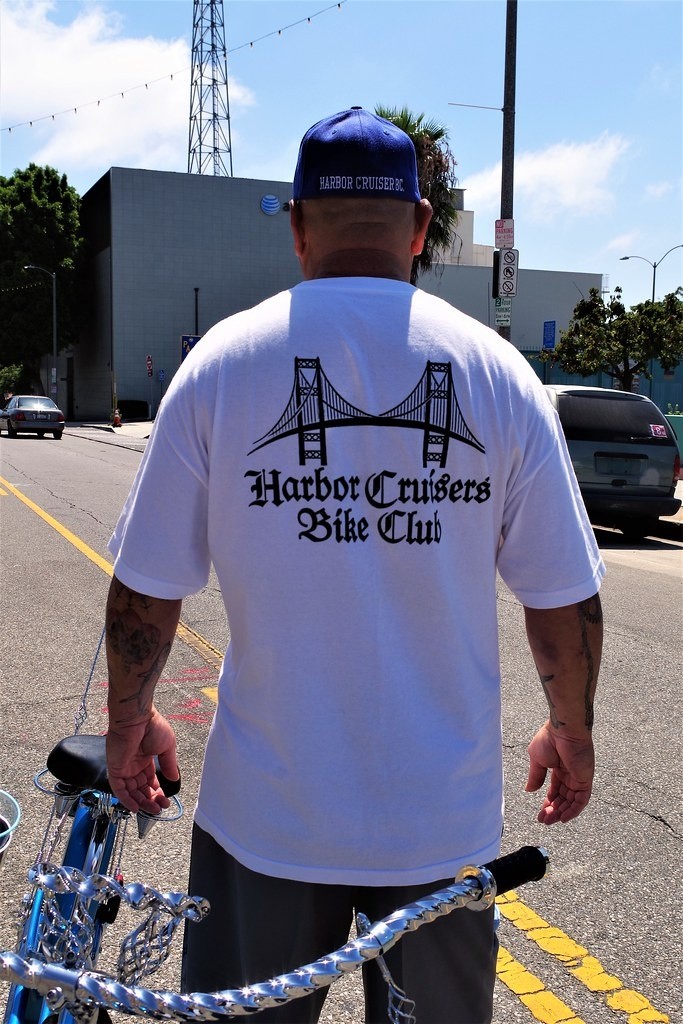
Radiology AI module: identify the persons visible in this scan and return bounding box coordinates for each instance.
[105,105,606,1024]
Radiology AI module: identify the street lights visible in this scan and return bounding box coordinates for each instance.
[25,265,57,404]
[621,243,683,396]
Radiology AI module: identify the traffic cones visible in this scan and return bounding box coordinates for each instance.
[112,408,121,427]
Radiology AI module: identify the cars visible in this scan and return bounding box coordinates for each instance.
[0,396,65,439]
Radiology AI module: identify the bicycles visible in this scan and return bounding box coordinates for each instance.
[0,734,552,1022]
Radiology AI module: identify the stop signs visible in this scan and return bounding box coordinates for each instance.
[146,355,152,368]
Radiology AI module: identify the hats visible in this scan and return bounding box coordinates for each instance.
[292,106,421,202]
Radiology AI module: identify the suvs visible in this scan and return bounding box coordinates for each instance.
[545,380,683,541]
